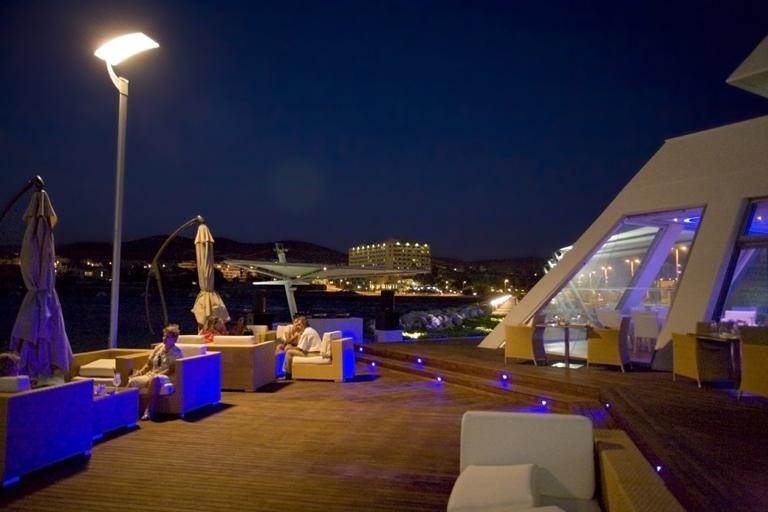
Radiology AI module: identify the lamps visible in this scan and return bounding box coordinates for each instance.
[93,31,160,347]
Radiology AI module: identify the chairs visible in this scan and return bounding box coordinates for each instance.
[447,410,687,512]
[737,326,768,400]
[671,322,734,389]
[505,303,659,374]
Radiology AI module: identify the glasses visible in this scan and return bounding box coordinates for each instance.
[162,334,176,339]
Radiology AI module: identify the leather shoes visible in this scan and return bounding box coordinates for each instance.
[278,372,291,380]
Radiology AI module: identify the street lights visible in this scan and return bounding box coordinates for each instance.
[443,280,466,295]
[600,266,613,288]
[502,278,509,294]
[89,30,163,352]
[576,270,596,288]
[623,258,639,278]
[669,246,686,282]
[339,279,342,288]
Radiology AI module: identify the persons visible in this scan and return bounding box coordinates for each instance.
[128,325,182,420]
[226,314,254,336]
[276,314,322,380]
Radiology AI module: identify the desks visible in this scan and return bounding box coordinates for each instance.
[93,385,138,439]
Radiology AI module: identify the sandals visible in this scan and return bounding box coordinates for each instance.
[141,411,155,420]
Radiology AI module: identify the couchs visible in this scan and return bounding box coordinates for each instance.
[120,348,222,419]
[65,349,148,386]
[150,326,354,393]
[1,376,94,485]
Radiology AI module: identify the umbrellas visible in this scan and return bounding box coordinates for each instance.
[10,176,74,378]
[190,224,232,335]
